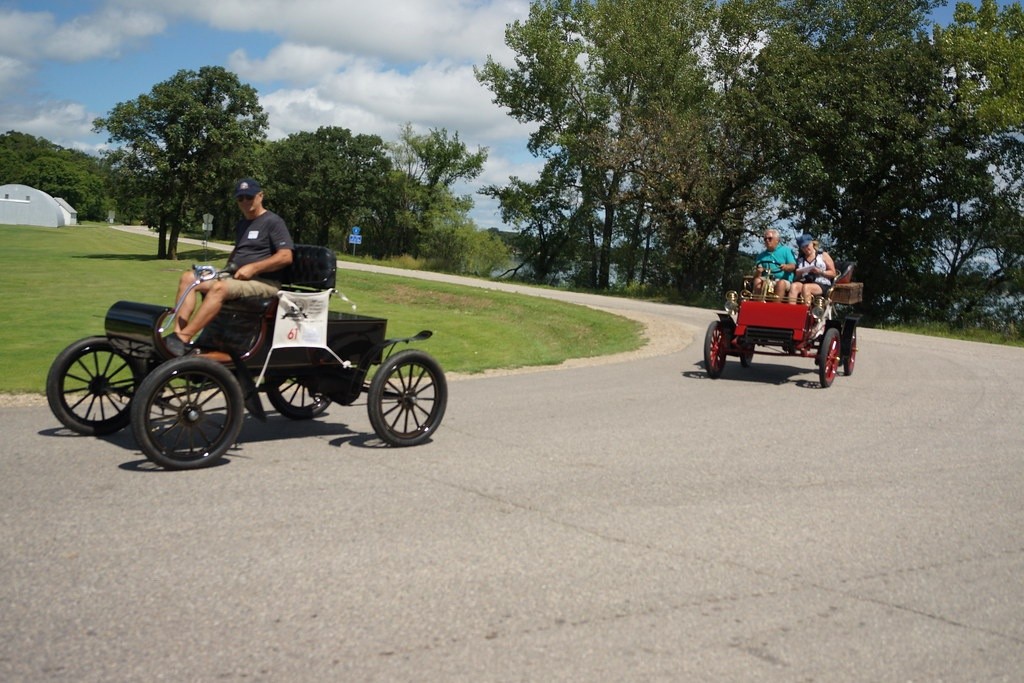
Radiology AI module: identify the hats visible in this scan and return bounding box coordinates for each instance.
[235,179,262,198]
[796,233,813,248]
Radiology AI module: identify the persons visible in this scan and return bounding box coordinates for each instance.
[789,235,836,314]
[162,179,294,355]
[753,229,797,302]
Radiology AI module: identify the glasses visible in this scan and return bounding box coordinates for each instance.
[763,237,772,242]
[237,195,255,202]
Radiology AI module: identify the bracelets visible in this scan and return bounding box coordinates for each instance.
[820,270,824,276]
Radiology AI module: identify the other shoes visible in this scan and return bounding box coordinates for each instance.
[162,332,185,358]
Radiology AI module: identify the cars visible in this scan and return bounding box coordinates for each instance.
[702,260,867,389]
[43,245,450,471]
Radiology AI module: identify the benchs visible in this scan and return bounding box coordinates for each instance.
[201,244,336,359]
[757,263,854,319]
